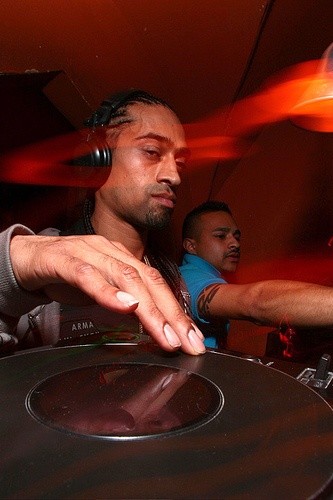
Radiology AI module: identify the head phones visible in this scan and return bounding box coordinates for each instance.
[66,88,144,188]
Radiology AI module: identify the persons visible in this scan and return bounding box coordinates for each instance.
[0,88,205,355]
[179,200,333,351]
